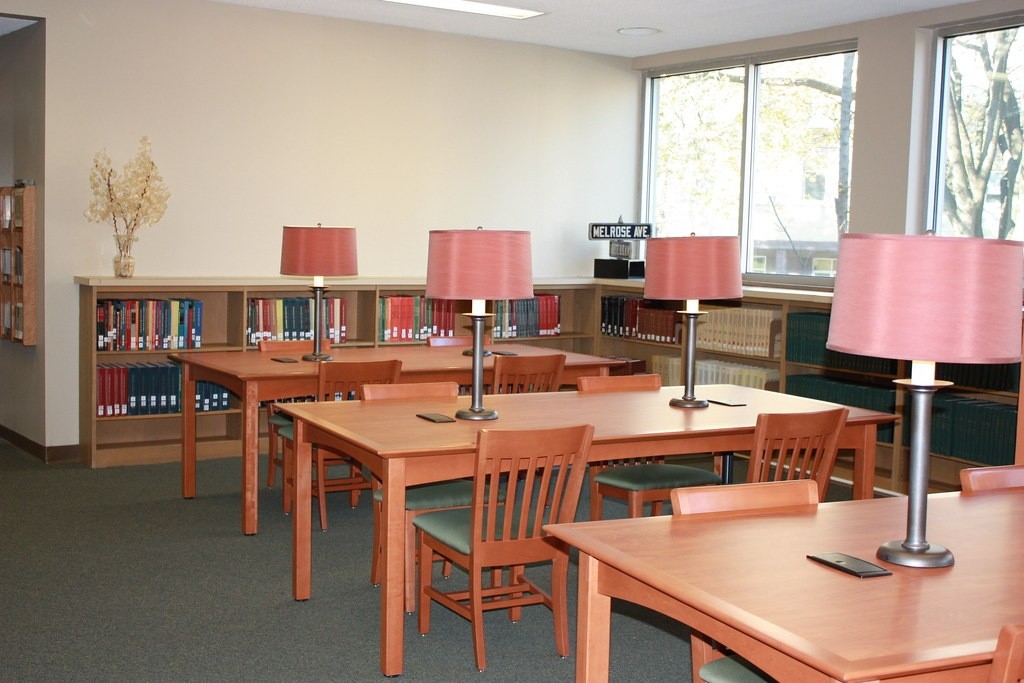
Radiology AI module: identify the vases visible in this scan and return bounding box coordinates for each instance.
[116,237,138,277]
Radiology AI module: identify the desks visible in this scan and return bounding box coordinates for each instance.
[543,484,1023,682]
[273,383,900,676]
[166,344,628,536]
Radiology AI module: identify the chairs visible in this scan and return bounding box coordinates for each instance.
[258,339,1024,683]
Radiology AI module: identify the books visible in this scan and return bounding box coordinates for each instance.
[785,374,896,443]
[96,298,202,351]
[905,361,1020,393]
[378,294,455,342]
[696,302,783,361]
[694,359,780,392]
[651,348,682,386]
[258,389,355,409]
[903,391,1018,466]
[786,312,897,375]
[247,297,346,346]
[0,187,24,339]
[601,295,683,345]
[96,359,230,419]
[494,294,560,339]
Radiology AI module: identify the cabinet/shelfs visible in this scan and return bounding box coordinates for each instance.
[74,275,1024,485]
[0,186,35,346]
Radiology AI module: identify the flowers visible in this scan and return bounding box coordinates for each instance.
[87,138,169,276]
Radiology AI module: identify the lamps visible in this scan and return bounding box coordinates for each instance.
[428,228,534,419]
[280,224,360,362]
[826,232,1024,568]
[645,233,744,408]
